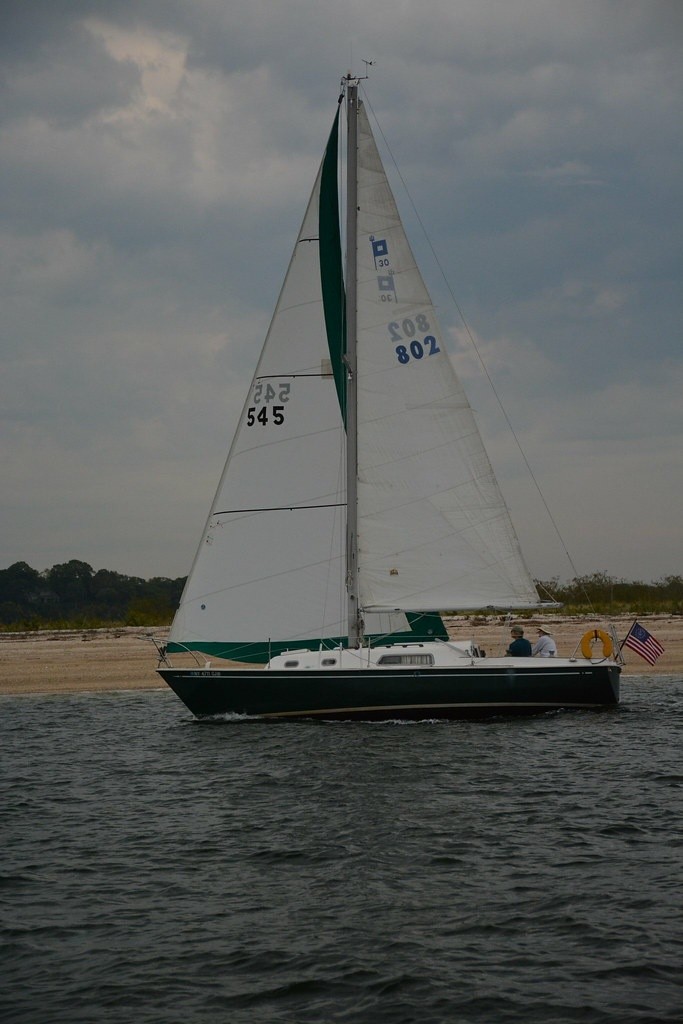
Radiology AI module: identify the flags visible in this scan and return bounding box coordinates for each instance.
[626,622,665,666]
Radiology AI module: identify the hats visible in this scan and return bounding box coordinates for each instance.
[510,626,523,632]
[536,625,553,635]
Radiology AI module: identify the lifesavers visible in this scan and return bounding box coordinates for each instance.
[580,630,611,657]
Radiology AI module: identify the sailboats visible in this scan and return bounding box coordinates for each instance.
[150,71,622,719]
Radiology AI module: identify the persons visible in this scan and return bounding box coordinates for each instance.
[532,625,557,657]
[503,625,531,657]
